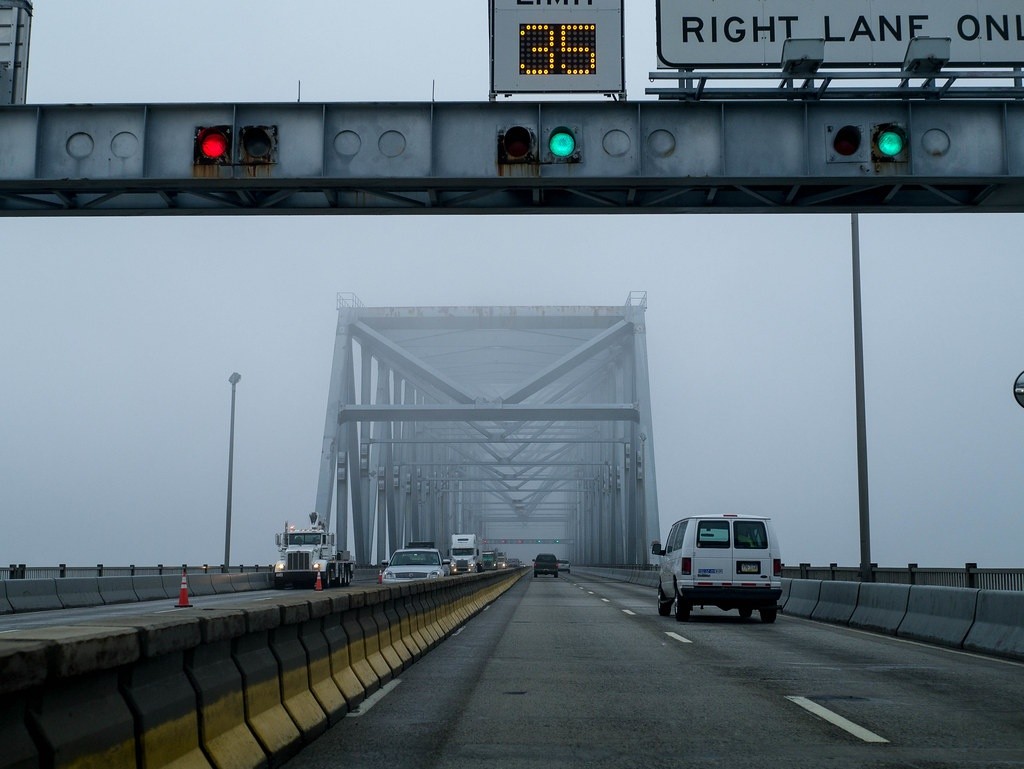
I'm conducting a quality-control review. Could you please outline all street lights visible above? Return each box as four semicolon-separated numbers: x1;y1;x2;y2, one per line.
221;371;243;573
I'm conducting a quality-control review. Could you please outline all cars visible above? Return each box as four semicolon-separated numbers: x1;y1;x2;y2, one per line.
1015;385;1024;394
557;559;572;573
380;540;451;584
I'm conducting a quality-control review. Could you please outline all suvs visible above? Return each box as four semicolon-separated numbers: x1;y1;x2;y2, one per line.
651;513;785;625
531;553;560;578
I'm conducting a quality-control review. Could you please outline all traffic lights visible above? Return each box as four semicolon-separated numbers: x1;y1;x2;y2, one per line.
193;123;280;166
824;119;910;164
497;121;584;165
488;0;626;95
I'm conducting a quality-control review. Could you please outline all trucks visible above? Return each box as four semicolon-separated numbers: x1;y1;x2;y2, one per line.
442;532;523;577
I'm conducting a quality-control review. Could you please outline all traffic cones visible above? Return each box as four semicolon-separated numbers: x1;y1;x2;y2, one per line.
377;569;383;584
173;571;194;608
313;570;323;591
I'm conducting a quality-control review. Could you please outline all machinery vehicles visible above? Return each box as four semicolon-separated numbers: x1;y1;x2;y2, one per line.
271;509;356;590
